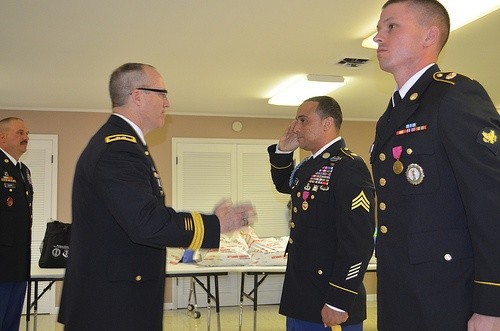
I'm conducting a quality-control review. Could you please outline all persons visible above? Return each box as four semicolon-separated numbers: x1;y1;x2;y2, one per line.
0;116;34;330
369;0;499;331
267;96;375;331
57;63;257;331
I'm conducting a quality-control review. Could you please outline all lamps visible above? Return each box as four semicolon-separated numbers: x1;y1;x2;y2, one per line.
267;75;344;106
362;0;500;53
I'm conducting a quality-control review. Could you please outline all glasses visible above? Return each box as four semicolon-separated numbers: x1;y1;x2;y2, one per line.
130;88;168;100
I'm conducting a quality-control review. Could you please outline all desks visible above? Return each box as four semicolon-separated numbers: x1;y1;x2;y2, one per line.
26;260;377;331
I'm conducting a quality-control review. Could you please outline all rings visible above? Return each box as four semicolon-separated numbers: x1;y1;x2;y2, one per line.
243;218;248;227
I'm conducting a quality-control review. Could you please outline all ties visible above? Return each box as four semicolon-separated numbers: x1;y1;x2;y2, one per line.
16;162;25;184
394;91;402;107
